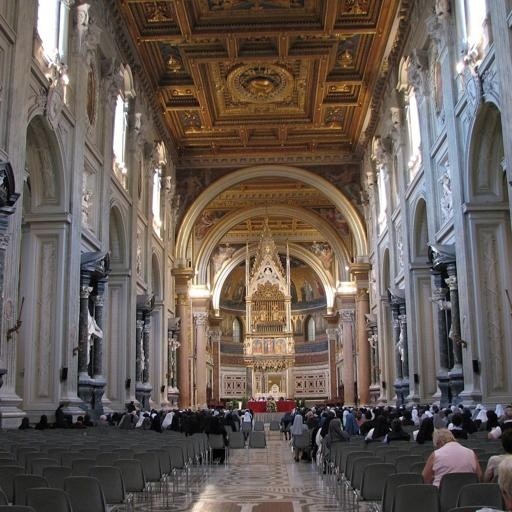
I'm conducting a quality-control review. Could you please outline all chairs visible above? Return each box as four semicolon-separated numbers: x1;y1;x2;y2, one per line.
269;403;511;511
0;404;266;512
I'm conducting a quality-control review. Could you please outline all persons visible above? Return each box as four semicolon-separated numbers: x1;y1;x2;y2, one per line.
281;402;512;512
18;404;253;465
